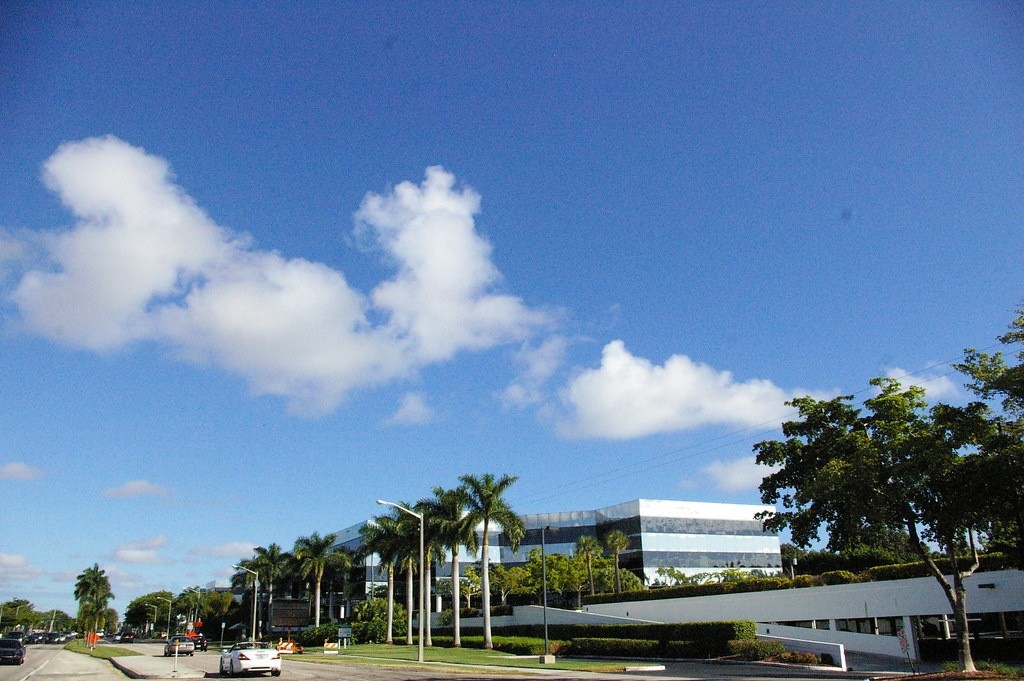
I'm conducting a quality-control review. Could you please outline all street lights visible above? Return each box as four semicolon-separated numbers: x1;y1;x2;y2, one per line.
182;587;200;631
157;597;171;641
539;525;560;663
145;604;157;623
376;500;424;663
232;565;258;641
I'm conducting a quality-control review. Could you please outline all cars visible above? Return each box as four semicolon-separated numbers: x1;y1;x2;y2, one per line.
0;639;27;665
112;634;122;641
219;641;282;677
119;633;134;643
191;636;207;652
164;636;194;657
2;631;79;645
